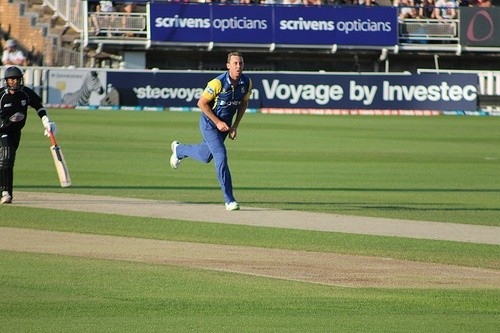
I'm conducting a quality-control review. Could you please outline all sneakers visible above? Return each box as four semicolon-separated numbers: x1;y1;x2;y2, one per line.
225;200;239;210
170;140;182;168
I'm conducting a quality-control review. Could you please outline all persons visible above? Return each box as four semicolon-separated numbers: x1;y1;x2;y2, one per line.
0;66;57;204
170;52;253;210
0;0;494;67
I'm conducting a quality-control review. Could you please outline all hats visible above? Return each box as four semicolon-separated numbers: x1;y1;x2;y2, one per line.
6;39;17;47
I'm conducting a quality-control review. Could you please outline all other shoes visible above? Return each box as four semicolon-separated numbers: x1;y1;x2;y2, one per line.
1;191;11;203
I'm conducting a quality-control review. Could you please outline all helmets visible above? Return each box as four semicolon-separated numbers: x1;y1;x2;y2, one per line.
3;67;23;79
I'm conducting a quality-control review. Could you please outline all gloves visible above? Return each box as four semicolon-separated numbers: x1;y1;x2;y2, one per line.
41;115;57;137
